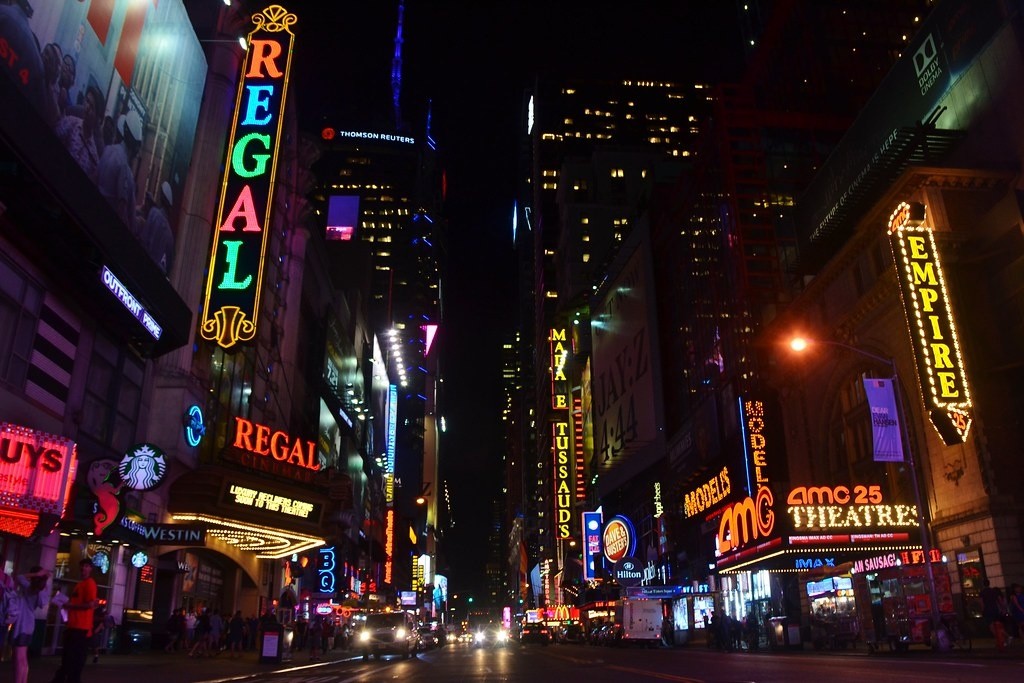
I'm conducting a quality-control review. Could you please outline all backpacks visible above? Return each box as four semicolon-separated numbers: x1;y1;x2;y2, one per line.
0;574;21;624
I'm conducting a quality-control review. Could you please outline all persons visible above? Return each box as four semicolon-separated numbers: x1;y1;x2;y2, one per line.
0;566;54;683
980;578;1024;651
59;558;115;683
710;602;773;652
164;605;352;661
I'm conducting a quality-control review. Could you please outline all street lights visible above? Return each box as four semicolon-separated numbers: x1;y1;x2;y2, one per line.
787;337;944;658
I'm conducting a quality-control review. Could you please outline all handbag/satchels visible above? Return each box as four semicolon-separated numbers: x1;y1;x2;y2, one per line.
61;628;88;644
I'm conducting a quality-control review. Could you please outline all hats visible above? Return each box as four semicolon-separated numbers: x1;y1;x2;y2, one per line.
79;558;93;567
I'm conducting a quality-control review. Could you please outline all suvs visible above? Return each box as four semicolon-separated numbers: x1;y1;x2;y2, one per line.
361;610;419;661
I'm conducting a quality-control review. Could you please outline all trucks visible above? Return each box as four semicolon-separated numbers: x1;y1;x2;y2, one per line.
616;596;664;646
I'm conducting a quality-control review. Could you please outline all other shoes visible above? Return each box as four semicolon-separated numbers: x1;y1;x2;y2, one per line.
93;655;98;664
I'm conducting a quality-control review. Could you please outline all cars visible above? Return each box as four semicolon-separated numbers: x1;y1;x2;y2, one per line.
556;626;588;644
590;622;620;645
520;623;549;647
471;623;505;648
415;619;469;653
